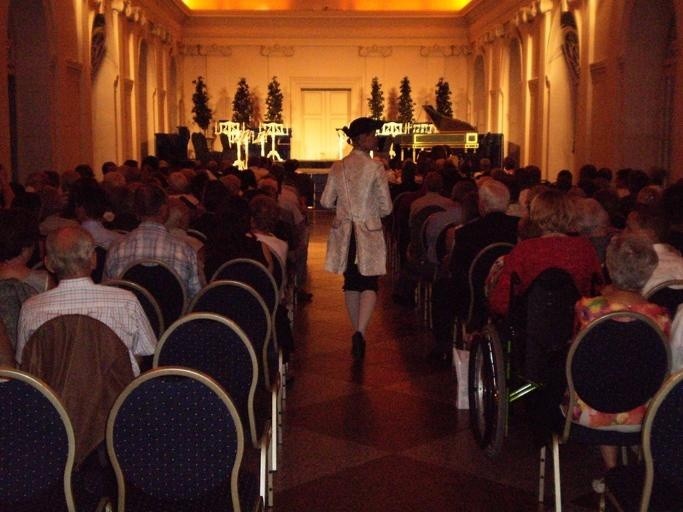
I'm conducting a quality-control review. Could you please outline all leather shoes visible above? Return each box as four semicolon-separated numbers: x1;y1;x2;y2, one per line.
352;332;366;356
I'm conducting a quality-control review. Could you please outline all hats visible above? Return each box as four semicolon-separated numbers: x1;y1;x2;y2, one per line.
350;118;383;135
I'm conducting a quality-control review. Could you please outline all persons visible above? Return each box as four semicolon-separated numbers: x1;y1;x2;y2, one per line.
447;163;680;486
3;154;313;315
370;147;496;271
319;117;393;356
16;222;158;382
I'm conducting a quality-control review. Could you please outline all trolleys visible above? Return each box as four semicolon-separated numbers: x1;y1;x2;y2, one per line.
466;267;585;455
